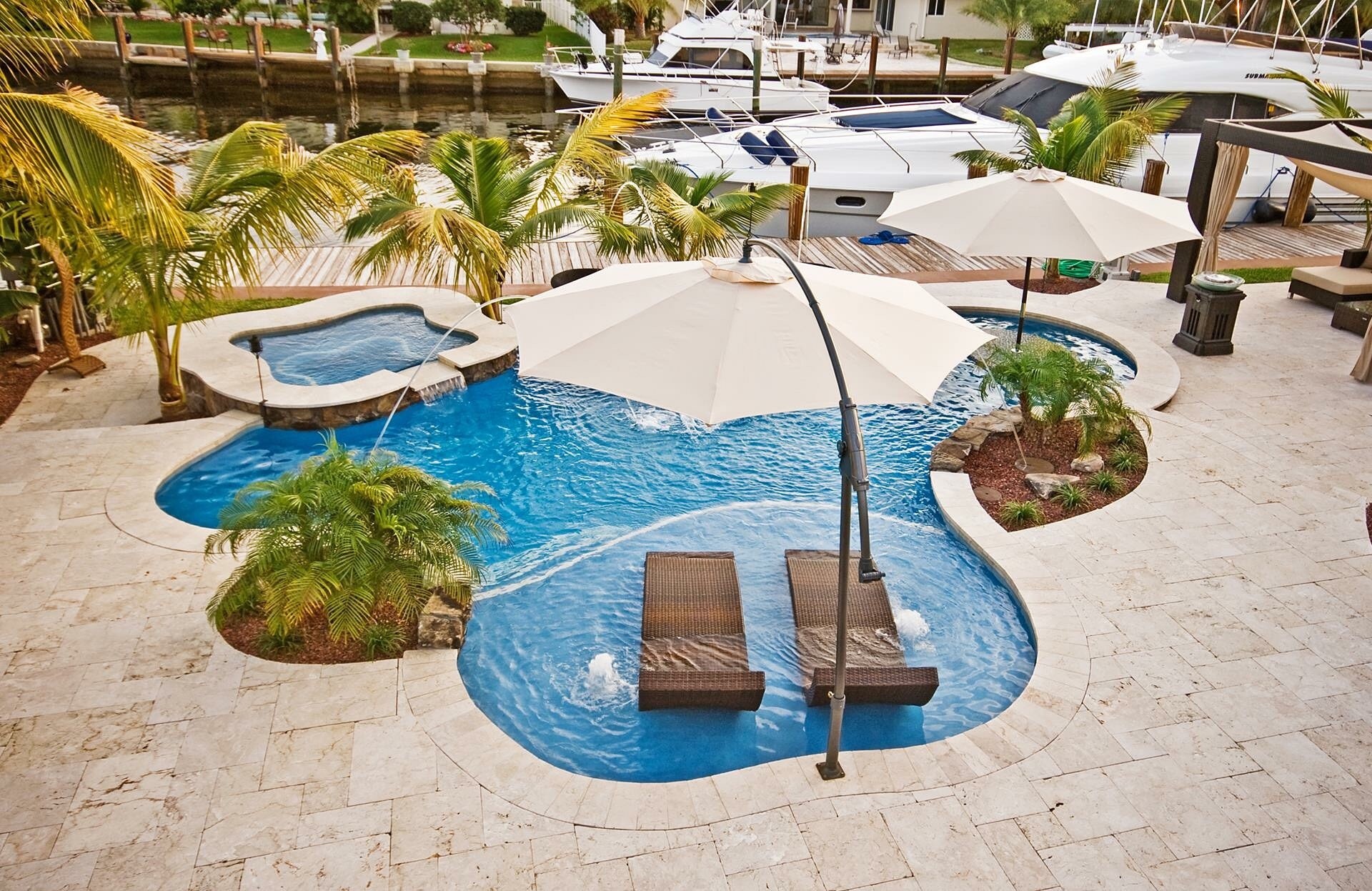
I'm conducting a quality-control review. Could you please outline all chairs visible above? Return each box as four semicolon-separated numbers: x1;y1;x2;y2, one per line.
637;551;765;710
826;43;846;64
784;10;798;29
888;35;913;59
246;31;271;55
858;21;891;43
204;28;233;52
846;39;866;64
785;548;940;708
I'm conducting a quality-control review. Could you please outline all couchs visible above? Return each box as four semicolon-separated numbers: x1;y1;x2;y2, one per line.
1289;248;1372;308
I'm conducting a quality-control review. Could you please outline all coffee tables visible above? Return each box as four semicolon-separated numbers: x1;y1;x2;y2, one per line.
1330;301;1372;335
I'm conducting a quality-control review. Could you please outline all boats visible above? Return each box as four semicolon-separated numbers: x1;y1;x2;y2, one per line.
549;18;842;116
622;21;1372;239
1042;23;1162;58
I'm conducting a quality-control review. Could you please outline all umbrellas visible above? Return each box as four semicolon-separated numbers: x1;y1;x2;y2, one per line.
877;161;1205;352
502;255;998;781
833;3;844;43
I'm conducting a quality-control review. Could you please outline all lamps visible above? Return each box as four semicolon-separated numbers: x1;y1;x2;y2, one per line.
248;335;263;355
